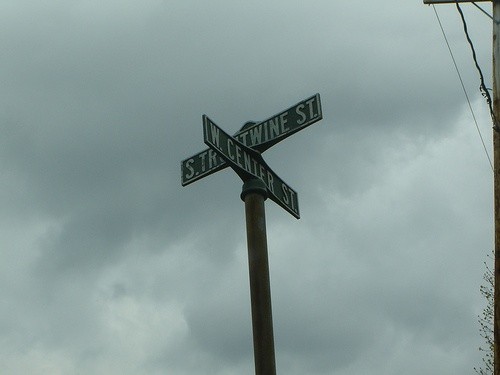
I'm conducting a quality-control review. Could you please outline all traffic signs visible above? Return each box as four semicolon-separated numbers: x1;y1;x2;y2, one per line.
202;112;300;220
179;91;323;189
